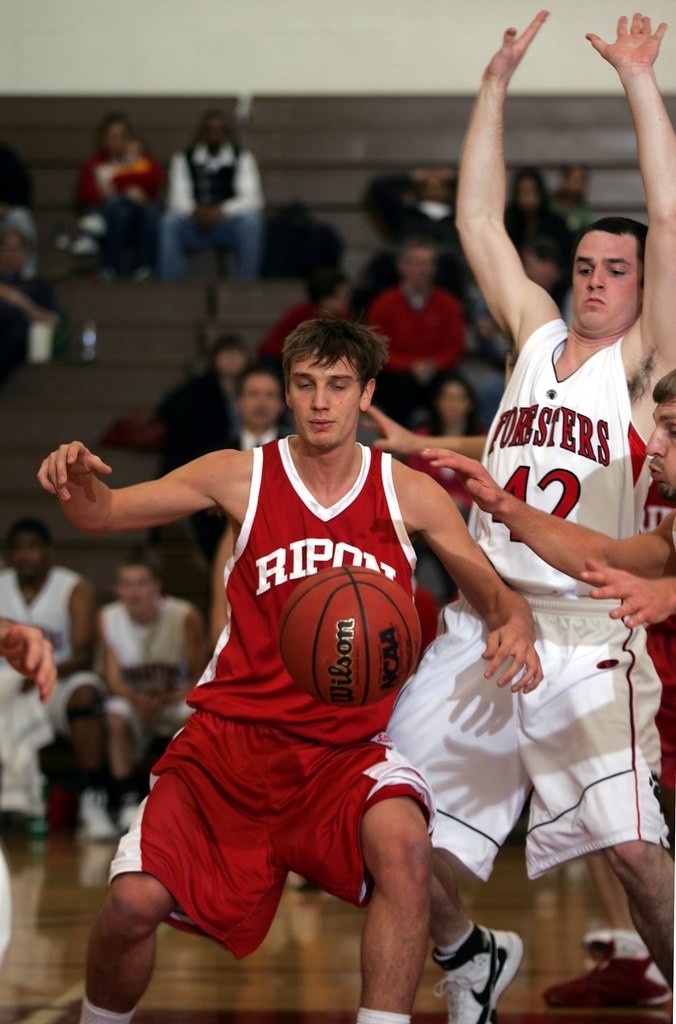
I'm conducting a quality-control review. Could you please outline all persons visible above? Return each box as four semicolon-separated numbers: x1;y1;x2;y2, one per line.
1;108;676;889
369;401;676;1009
386;10;676;1024
418;366;676;855
36;321;544;1024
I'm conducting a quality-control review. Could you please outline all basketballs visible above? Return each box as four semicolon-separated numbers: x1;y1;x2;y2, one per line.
275;565;423;710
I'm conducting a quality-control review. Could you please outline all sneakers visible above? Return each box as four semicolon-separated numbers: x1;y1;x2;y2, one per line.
431;924;524;1024
542;934;673;1006
